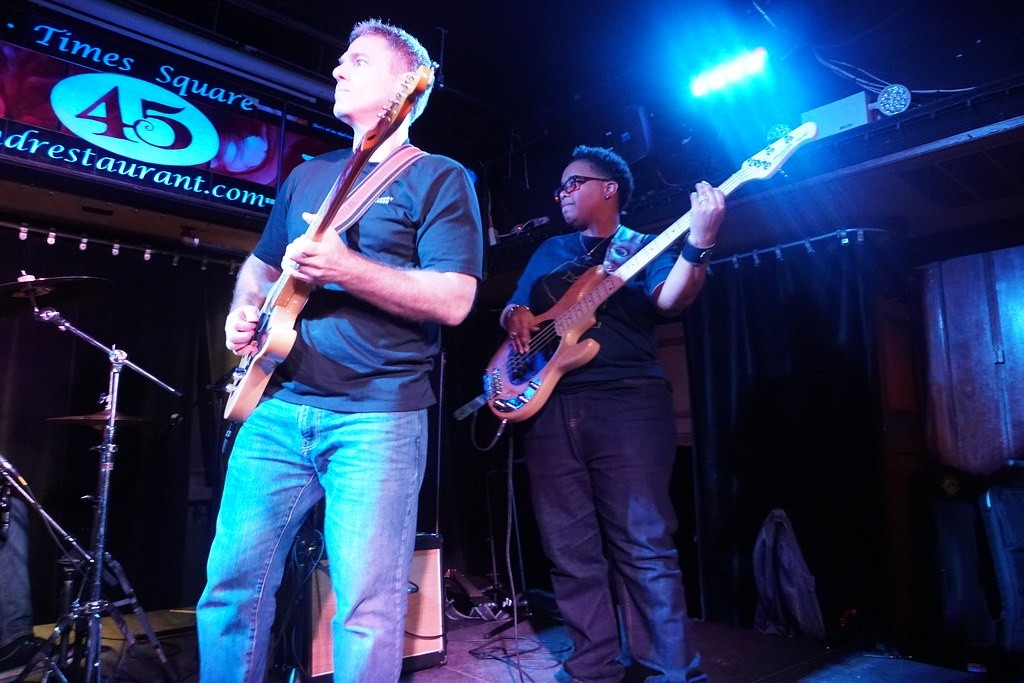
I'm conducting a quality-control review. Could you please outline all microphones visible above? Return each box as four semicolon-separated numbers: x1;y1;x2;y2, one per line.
0;455;17;476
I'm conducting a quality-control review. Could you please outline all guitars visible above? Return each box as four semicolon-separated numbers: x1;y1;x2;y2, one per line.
223;64;431;423
483;121;817;423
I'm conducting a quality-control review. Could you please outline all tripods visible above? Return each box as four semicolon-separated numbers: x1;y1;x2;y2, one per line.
482;487;560;638
11;287;181;682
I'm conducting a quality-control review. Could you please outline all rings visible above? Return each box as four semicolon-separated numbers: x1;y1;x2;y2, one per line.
509;332;517;338
294;263;301;271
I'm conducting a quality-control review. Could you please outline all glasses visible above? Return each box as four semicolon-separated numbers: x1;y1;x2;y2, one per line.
552;175;620;203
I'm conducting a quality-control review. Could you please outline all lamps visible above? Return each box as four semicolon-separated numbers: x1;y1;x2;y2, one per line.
766;83;913;146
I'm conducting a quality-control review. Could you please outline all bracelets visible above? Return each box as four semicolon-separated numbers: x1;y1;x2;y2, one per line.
507;304;529;317
682;232;717;266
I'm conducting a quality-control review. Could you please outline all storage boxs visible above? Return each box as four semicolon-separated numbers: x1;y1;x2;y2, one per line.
274;533;446;683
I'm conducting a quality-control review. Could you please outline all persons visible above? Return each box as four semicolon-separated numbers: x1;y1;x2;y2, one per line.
193;18;484;683
0;498;119;683
486;142;725;683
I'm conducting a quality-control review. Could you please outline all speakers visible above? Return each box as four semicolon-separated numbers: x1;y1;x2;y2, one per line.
274;531;449;683
1;485;28;658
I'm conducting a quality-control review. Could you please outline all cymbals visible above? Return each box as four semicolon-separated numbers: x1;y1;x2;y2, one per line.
0;276;116;299
41;408;162;433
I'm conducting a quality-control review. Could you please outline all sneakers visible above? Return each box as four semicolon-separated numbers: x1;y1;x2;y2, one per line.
0;634;75;683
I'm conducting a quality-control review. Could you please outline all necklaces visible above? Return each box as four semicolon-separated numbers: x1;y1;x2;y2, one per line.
579;224;623;260
